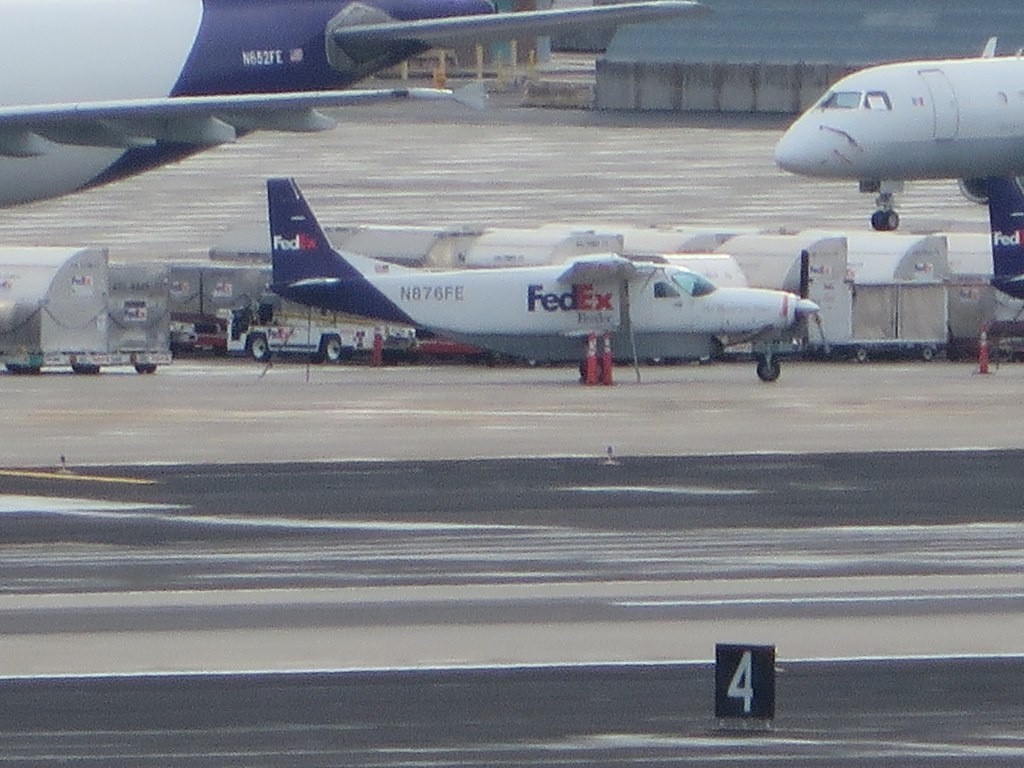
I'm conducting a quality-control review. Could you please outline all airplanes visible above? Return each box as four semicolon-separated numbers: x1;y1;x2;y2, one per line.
772;36;1024;231
264;177;820;383
0;0;712;202
